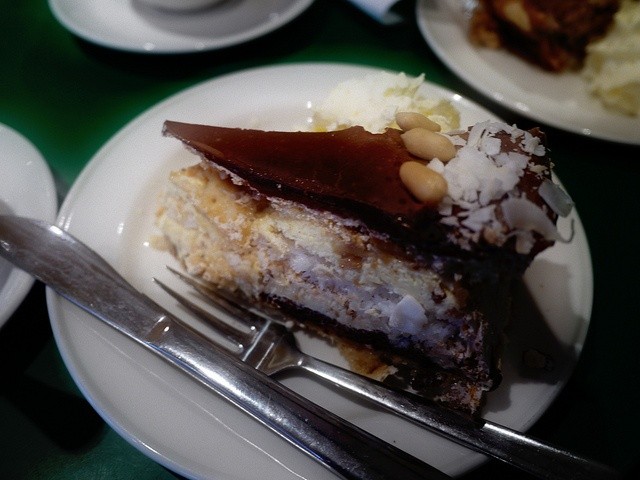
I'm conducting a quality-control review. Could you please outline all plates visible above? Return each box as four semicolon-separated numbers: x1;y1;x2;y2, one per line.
417;2;639;145
54;0;310;53
2;127;59;335
45;60;594;479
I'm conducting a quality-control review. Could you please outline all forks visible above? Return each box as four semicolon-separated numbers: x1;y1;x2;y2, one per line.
137;263;608;478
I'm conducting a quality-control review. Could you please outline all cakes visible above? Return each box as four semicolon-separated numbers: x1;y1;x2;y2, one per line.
149;71;573;422
468;0;640;117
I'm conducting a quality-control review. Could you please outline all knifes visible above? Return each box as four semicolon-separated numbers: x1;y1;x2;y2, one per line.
0;215;455;479
